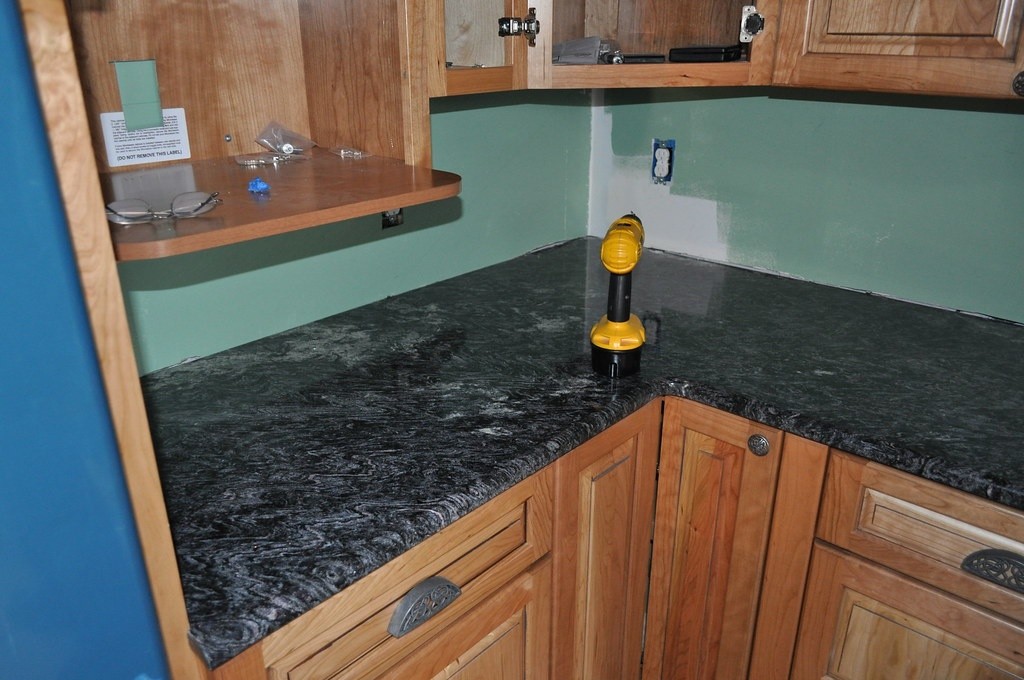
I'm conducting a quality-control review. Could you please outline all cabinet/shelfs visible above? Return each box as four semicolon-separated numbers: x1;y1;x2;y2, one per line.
63;0;1023;265
204;398;1024;679
